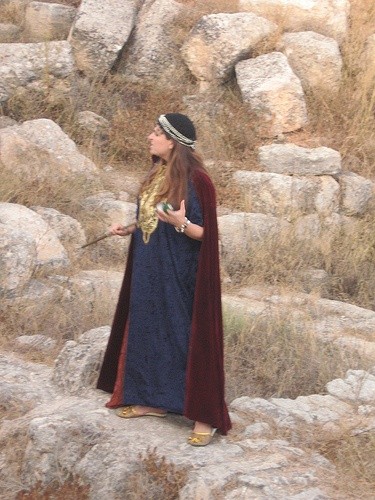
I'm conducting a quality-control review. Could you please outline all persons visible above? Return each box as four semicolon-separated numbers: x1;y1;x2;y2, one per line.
96;113;233;446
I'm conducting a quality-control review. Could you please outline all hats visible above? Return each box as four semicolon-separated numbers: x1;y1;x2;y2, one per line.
158;113;197;149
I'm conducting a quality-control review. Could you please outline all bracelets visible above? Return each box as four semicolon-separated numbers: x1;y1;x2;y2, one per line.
174;217;190;233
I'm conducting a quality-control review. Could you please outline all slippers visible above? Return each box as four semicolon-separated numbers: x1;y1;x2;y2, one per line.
116;405;168;418
187;428;218;446
